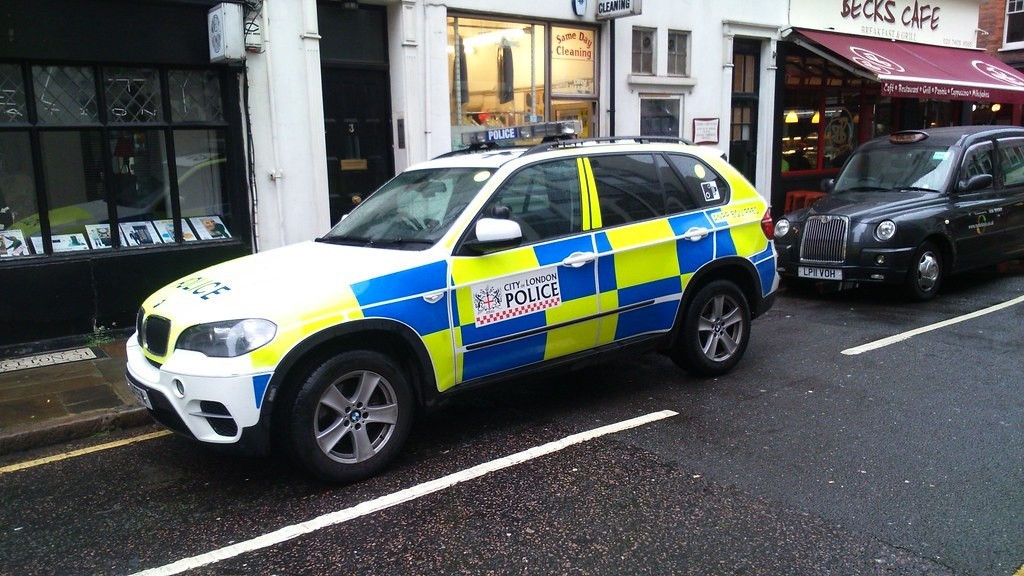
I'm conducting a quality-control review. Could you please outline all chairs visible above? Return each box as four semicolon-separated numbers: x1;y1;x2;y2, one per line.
544;165;685;236
968;161;987;178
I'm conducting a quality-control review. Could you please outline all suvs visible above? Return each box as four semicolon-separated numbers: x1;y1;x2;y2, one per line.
6;139;391;256
772;123;1023;301
122;119;780;483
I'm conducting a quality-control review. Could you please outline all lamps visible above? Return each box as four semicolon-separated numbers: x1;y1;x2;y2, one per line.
785;110;798;123
811;111;819;123
